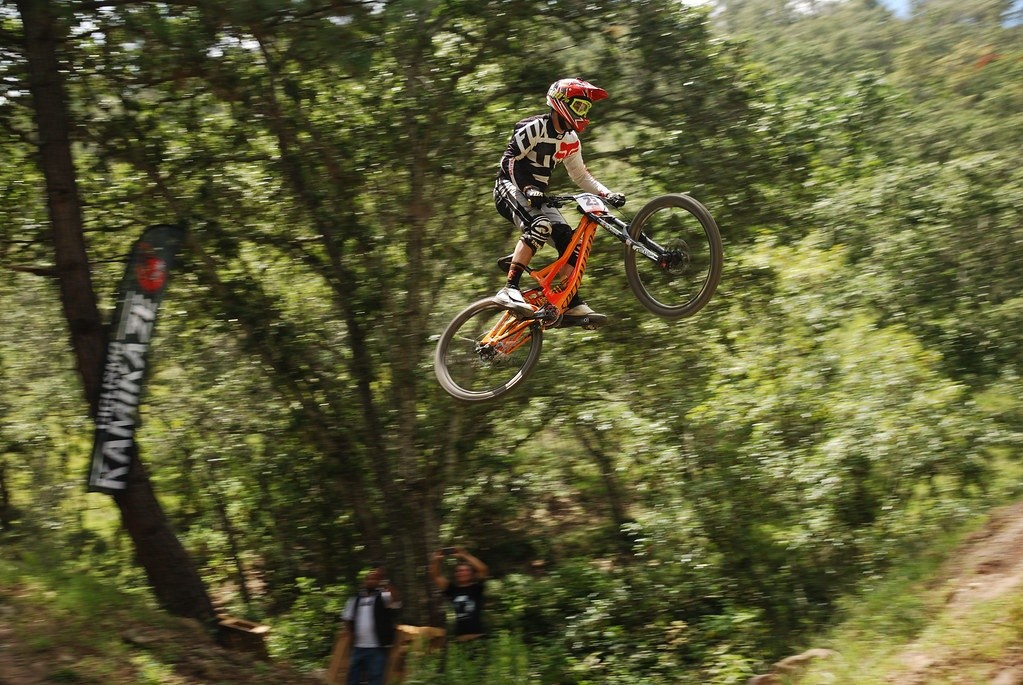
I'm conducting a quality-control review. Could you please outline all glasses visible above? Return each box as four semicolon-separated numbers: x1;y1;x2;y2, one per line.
568;98;592;118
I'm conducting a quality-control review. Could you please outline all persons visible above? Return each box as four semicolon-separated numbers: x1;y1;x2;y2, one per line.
340;566;402;685
430;545;488;661
492;77;626;328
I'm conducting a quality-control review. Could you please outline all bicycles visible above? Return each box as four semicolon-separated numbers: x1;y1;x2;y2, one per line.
433;188;722;405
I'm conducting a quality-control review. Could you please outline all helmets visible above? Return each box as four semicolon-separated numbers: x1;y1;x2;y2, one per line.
546;78;608;133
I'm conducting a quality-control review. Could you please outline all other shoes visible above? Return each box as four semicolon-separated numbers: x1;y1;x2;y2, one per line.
563;303;608;325
493;284;534;318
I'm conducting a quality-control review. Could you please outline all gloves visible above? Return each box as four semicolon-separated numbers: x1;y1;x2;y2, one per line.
524;187;545;209
606;192;626;207
